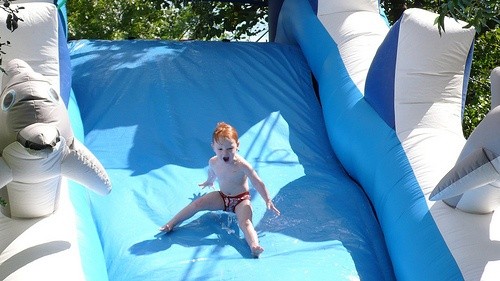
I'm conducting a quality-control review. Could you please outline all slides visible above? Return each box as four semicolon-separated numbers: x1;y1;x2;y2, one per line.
68;40;394;280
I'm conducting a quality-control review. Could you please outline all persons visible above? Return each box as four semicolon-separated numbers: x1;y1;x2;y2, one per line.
159;122;280;258
